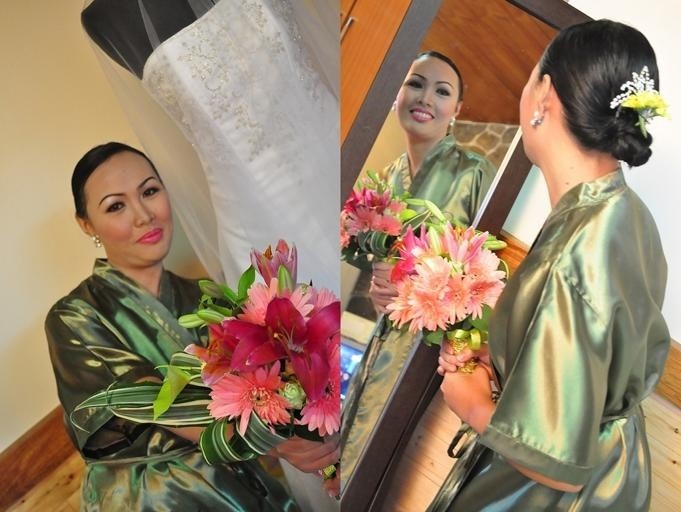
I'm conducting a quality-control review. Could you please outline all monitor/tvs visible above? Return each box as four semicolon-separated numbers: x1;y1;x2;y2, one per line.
340;335;368;401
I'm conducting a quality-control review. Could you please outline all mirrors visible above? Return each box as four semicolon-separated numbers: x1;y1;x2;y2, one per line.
339;1;561;507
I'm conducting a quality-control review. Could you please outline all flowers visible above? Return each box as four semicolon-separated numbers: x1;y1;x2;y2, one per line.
69;238;340;499
384;199;509;375
339;167;425;265
620;89;673;139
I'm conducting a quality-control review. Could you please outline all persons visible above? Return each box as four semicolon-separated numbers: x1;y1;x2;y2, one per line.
424;19;671;512
340;50;497;503
44;141;340;512
80;0;340;301
